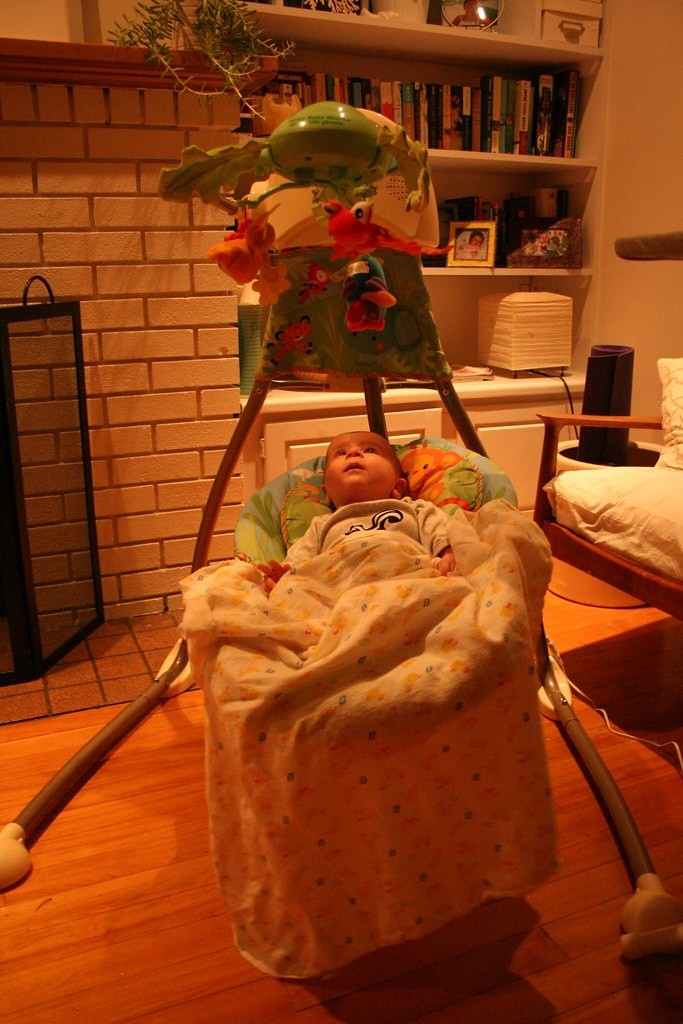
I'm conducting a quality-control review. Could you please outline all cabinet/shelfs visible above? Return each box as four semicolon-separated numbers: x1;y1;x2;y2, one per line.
253;391;584;521
216;0;605;414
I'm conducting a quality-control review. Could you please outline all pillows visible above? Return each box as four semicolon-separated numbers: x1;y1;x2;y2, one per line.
654;358;683;472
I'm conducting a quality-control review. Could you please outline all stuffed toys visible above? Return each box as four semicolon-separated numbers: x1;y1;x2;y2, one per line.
207;202;290;305
376;122;430;213
320;197;395;333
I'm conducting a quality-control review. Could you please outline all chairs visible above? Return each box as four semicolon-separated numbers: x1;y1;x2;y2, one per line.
532;413;683;622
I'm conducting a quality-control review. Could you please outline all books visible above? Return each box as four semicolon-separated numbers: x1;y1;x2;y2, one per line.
424;186;568;265
238;63;582;157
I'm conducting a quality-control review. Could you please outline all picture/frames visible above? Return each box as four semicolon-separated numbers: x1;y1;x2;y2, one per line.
446;220;498;268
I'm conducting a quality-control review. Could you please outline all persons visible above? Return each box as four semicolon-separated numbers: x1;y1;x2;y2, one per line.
257;430;456;594
451;0;492;26
459;231;486;259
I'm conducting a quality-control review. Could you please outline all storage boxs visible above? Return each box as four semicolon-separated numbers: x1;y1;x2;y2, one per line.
542;0;604;48
